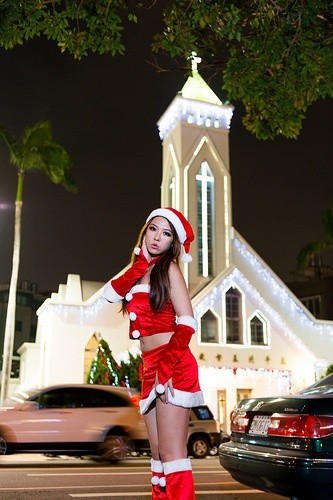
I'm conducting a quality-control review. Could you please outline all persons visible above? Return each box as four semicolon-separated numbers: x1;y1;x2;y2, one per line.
104;208;204;500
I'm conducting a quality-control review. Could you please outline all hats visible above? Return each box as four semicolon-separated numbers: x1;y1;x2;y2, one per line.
146;207;194;263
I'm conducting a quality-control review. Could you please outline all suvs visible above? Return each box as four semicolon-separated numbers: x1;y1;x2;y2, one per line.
0;383;141;463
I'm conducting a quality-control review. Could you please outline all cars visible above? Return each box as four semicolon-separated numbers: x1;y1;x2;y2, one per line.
218;373;333;500
132;405;219;459
